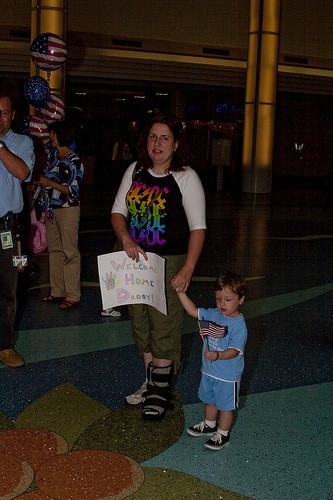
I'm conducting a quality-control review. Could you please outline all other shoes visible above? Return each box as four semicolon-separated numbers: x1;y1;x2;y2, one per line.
0;349;25;367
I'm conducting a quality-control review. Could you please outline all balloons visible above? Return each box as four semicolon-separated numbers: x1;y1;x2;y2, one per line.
30;33;68;71
19;76;65;146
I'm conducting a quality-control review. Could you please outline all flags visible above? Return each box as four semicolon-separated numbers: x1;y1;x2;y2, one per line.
199;321;225;338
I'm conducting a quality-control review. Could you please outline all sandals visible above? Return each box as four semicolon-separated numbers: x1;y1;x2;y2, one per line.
58;299;79;310
101;308;121;316
42;296;65;302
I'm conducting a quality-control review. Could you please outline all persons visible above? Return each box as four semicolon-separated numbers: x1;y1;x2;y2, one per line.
111;116;207;419
36;121;85;310
0;93;36;368
100;239;123;317
171;272;248;449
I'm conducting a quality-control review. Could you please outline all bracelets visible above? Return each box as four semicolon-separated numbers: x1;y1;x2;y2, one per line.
214;351;219;361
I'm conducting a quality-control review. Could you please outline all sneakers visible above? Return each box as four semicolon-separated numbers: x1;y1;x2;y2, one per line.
204;431;230;450
187;422;218;436
124;382;149;404
